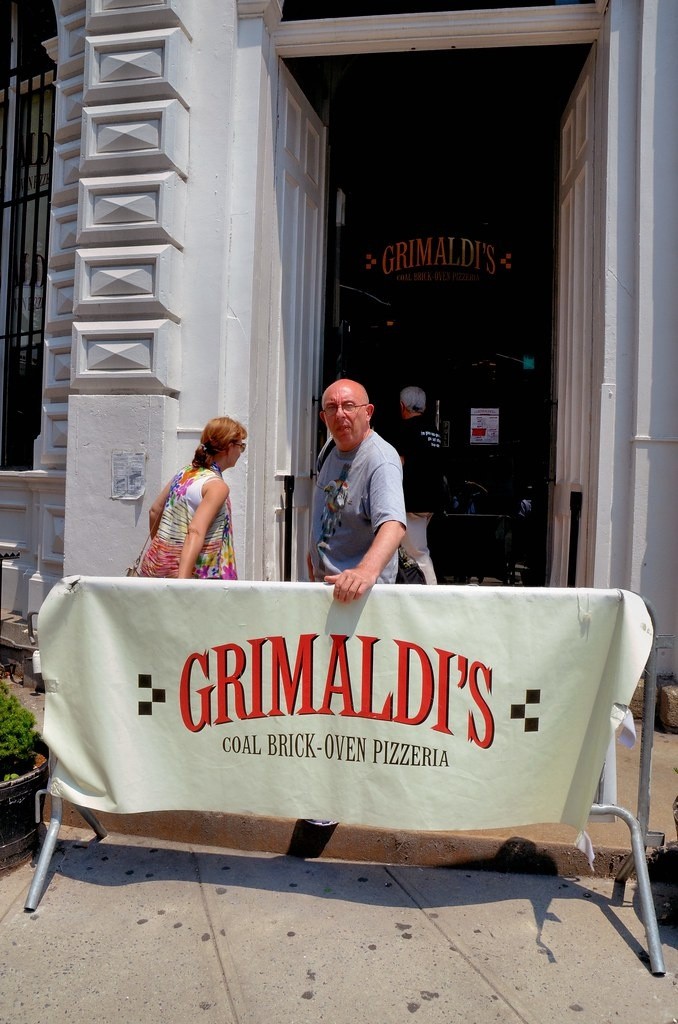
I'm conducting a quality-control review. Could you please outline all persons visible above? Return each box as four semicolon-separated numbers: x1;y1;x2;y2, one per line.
303;379;407;826
397;386;442;586
140;418;247;579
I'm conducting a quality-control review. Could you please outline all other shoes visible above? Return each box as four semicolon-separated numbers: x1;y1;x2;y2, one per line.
305;819;337;827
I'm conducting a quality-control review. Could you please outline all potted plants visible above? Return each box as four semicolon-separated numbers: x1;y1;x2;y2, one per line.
0;681;49;869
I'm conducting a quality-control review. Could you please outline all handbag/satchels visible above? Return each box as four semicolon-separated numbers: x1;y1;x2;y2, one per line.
393;554;427;585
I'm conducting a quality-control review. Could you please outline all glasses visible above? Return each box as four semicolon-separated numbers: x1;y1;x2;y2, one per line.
323;402;369;416
231;441;247;452
